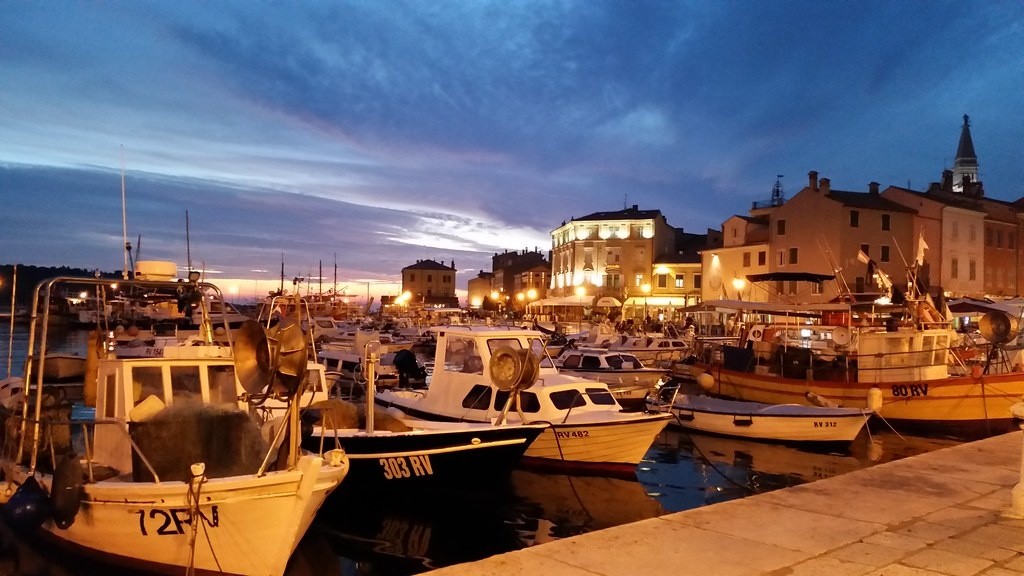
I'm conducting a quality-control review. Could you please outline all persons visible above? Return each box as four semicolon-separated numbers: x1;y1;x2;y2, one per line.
618;311;699;335
393;349;417;388
884;312;898;332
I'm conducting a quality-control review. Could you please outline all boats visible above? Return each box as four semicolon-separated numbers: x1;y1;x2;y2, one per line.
127;210;249;328
567;333;690;361
638;384;882;448
679;294;1024;433
371;325;681;480
274;346;554;556
254;253;363;326
0;169;349;576
551;347;674;403
309;315;419;366
